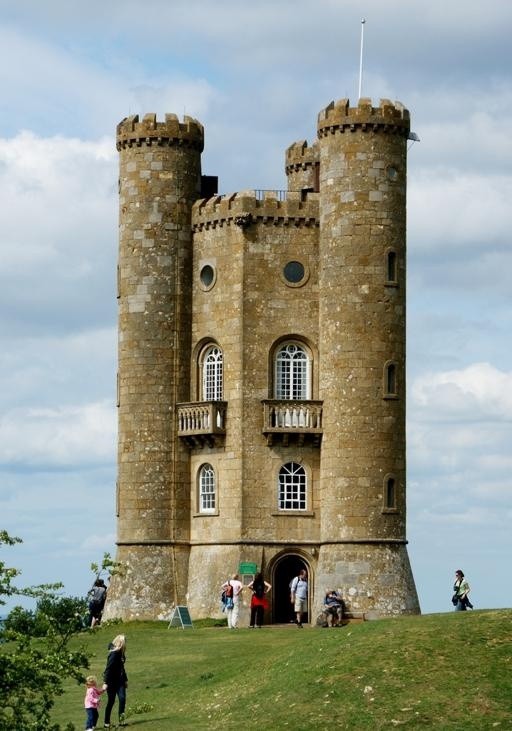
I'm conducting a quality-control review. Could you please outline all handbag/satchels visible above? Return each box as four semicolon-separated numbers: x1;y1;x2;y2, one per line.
223;579;234;598
452;595;458;606
288;576;299;593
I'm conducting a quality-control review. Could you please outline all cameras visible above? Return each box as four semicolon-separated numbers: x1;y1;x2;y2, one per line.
453;585;459;591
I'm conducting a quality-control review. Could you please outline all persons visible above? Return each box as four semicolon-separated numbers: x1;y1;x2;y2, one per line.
289;570;308;629
83;676;106;731
102;634;129;730
222;574;244;630
453;570;470;612
85;581;107;627
89;580;106;631
324;590;345;628
246;572;272;628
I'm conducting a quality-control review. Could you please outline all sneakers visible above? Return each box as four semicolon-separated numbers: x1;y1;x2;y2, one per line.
225;620;345;630
